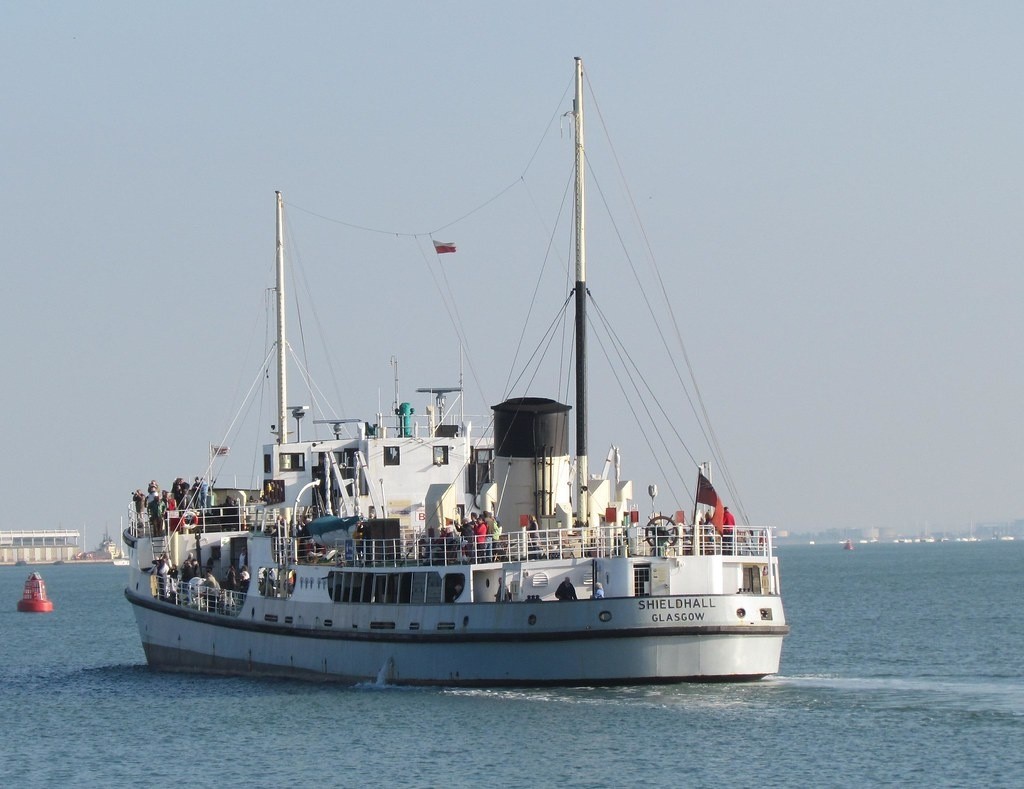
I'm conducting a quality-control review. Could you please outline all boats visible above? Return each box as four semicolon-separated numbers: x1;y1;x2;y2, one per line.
123;53;790;685
306;515;359;546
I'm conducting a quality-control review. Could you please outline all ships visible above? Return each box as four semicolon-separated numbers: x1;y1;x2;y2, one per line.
1;515;130;567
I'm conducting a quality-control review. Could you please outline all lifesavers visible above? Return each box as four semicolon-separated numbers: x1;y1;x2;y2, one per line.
307;538;327;557
462;540;472;562
181;511;198;529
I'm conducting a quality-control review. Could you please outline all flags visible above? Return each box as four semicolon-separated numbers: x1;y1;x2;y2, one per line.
697;473;723;537
432;240;456;254
211;445;230;456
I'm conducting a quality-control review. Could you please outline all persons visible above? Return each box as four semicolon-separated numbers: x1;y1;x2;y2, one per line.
238;547;248;576
290;513;375;563
200;477;208;513
226;566;236;606
445;526;453;564
144;475;191;537
152;551;200;606
192;476;201;511
238;565;250;592
274;514;286;556
722;507;734;555
528;513;540;561
199;569;220;612
455;518;473;564
468;511;499;563
594;582;604;599
259;568;276;597
133;488;147;528
555;577;577;600
